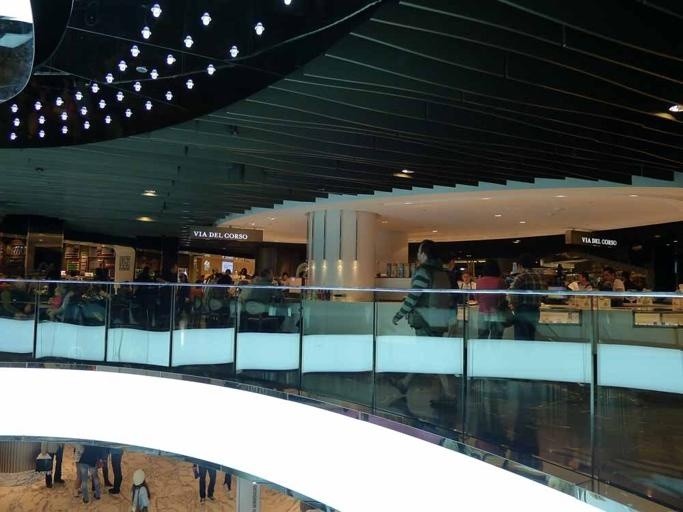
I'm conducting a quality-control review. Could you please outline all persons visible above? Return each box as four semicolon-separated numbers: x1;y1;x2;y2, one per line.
222;472;234;500
130;469;150;512
0;267;291;330
34;440;124;504
192;463;216;505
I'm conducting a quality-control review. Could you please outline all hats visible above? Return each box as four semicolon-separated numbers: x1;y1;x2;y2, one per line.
133;469;145;486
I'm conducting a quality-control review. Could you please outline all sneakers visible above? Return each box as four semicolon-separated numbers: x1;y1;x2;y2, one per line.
200;496;215;504
430;396;457;406
389;376;408;394
74;480;120;503
46;479;65;488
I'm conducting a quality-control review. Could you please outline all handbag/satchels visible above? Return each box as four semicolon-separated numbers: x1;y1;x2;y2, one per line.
497;302;514;327
35;453;52;473
